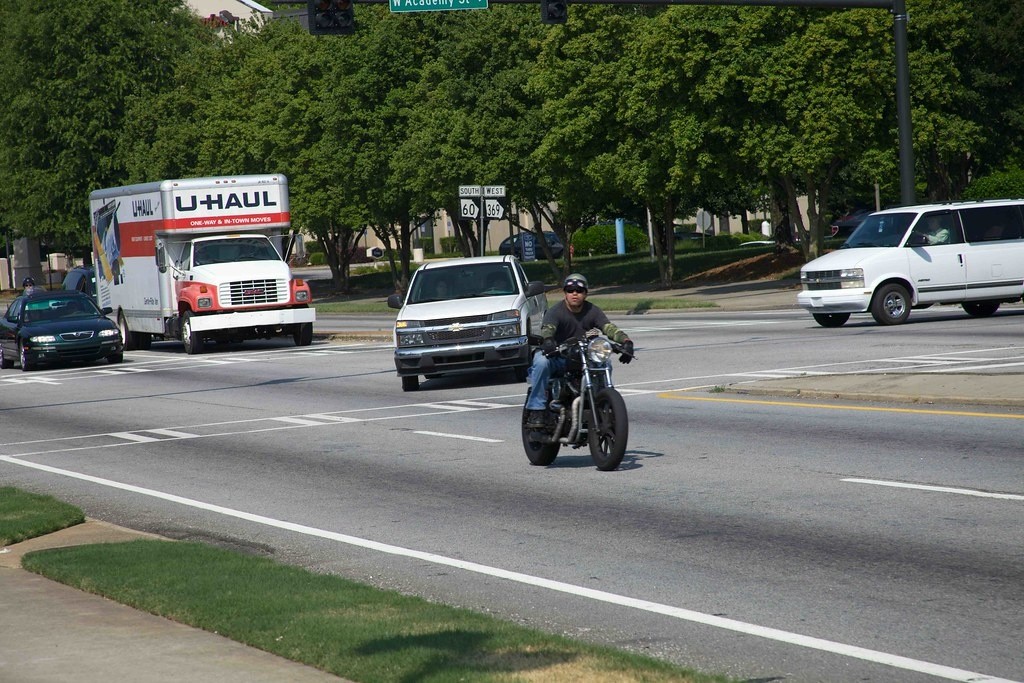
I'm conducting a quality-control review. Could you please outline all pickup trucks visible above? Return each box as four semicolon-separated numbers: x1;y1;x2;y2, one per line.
386;253;550;392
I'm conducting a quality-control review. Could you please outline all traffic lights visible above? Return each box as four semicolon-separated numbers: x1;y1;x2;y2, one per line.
307;0;354;36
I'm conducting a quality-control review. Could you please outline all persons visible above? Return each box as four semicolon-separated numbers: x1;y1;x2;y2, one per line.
916;217;950;245
526;272;634;427
17;277;37;297
435;279;448;297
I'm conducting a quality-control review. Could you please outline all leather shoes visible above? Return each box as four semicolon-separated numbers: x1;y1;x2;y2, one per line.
523;411;545;429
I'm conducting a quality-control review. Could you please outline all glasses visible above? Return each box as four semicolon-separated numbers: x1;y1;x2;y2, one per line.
25;285;32;287
564;288;585;294
565;279;586;287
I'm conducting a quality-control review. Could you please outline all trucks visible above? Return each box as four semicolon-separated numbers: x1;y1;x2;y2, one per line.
88;171;316;355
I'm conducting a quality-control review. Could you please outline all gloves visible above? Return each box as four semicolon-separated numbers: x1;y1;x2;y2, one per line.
540;338;557;352
619;341;634;364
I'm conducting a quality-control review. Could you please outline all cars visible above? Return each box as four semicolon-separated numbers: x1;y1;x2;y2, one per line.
58;266;96;304
0;287;124;371
499;230;565;260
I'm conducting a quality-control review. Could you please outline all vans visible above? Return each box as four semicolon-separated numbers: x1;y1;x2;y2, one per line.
796;197;1024;326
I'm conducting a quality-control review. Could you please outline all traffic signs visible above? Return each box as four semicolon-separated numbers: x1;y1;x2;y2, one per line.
389;0;488;13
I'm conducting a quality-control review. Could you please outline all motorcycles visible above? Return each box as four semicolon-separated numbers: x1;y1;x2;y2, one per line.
520;335;639;471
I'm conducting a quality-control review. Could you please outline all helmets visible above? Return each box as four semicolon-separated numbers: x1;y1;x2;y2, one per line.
563;274;588;293
22;277;35;287
